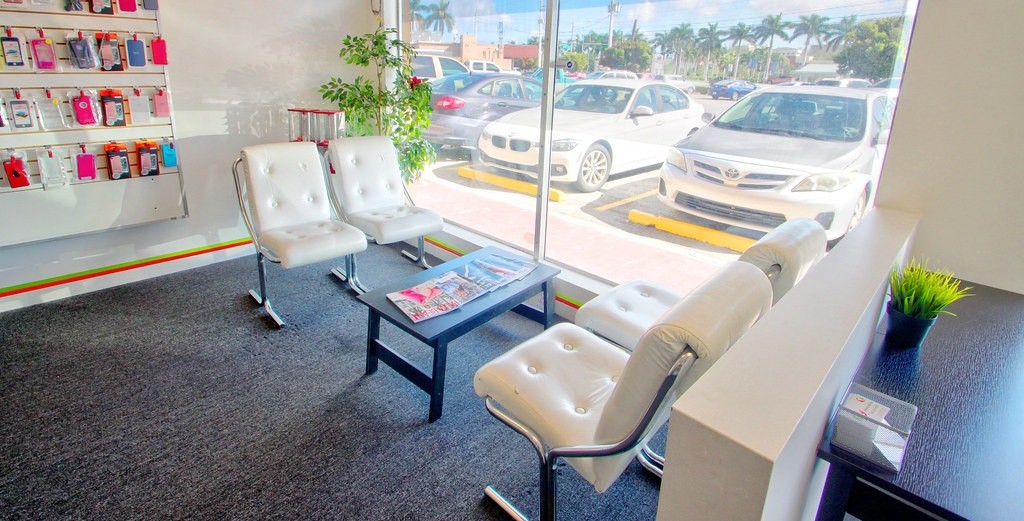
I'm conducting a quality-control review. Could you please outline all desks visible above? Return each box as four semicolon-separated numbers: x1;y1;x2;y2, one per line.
356;246;563;422
815;270;1024;521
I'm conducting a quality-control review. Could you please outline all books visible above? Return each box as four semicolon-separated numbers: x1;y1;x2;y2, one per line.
385;253;539;324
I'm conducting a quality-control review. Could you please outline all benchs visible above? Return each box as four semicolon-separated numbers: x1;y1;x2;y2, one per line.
234;135;445;329
474;214;829;521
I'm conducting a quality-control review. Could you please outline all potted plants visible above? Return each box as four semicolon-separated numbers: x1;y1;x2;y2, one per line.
885;257;974;348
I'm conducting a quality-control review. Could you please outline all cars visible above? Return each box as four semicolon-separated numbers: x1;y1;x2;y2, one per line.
409;55;705;193
657;75;902;247
